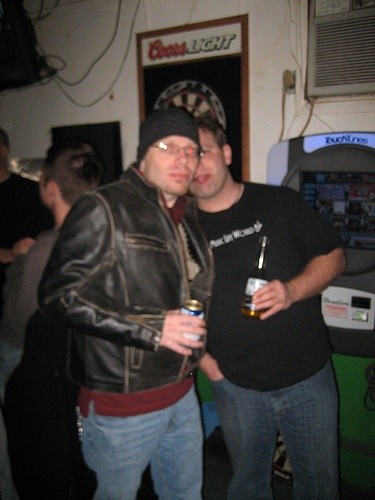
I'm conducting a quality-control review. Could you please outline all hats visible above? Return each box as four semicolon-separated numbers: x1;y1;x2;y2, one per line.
139;108;200;148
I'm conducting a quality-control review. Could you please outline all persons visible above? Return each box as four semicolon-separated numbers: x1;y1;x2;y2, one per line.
0;127;56;293
184;114;346;500
0;139;103;500
0;403;20;500
35;105;213;500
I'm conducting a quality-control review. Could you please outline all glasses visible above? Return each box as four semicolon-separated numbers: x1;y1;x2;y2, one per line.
148;141;200;158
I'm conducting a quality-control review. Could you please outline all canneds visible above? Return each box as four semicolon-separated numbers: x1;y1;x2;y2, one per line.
180;299;205;341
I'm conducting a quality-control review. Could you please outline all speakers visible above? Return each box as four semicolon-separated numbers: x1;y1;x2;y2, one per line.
0;0;40;92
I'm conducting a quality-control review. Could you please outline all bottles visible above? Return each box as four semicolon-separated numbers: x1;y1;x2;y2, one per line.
242;234;276;319
